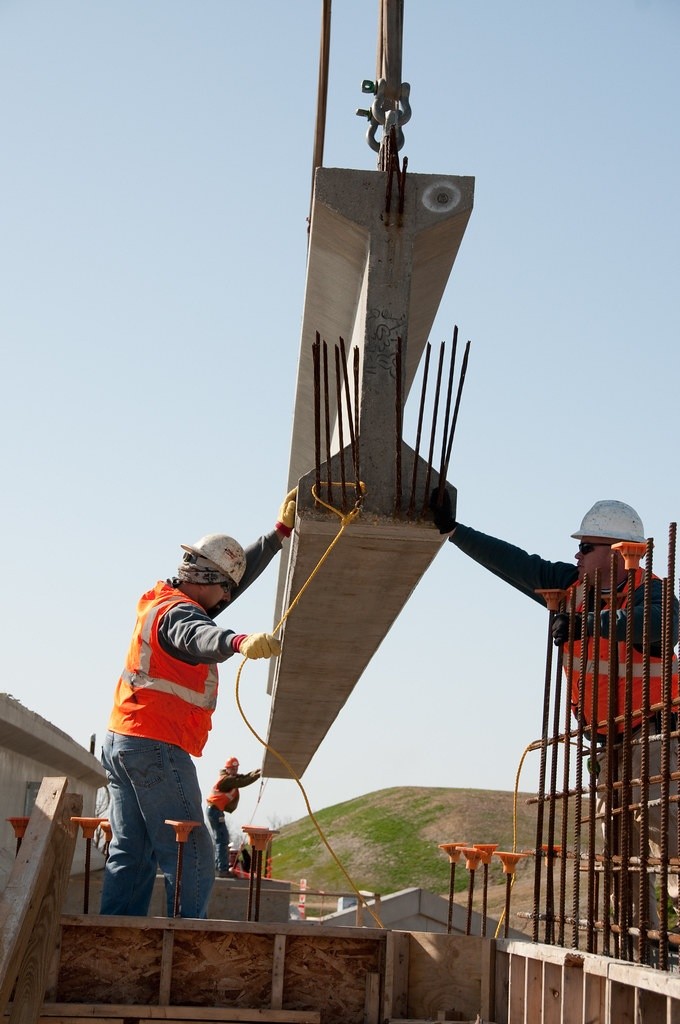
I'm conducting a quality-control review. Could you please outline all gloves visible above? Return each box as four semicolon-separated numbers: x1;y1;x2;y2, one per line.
274;485;298;538
234;633;282;659
550;613;590;646
431;487;457;534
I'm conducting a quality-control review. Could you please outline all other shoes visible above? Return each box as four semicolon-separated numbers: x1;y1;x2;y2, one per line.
215;869;234;877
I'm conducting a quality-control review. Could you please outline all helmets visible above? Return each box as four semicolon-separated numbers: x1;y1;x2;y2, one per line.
225;757;238;768
571;501;647;542
181;533;247;585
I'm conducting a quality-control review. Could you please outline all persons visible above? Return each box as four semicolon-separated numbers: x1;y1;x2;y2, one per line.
448;500;680;965
228;842;251;880
98;485;298;918
207;757;261;878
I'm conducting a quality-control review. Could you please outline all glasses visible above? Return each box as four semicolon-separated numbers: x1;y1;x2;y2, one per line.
578;542;613;555
